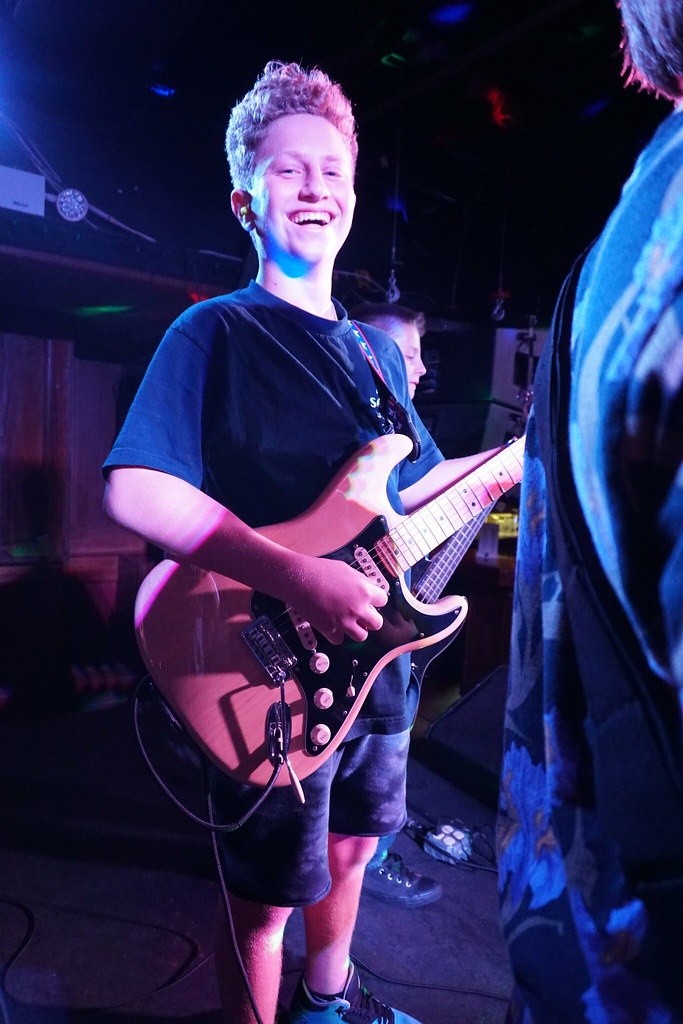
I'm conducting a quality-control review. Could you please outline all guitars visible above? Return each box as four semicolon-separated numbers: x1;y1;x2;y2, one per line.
126;428;526;810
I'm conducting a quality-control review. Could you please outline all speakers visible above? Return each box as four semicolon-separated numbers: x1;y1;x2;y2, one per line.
412;329;550;505
422;663;508;812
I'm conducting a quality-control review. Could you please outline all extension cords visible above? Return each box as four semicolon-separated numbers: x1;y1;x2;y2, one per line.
425;824;473;865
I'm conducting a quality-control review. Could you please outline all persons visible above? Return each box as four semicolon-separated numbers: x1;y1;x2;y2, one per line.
98;53;461;1024
342;300;485;911
490;2;683;1022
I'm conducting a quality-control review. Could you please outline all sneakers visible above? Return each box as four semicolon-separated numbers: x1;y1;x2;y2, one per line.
362;851;442;907
289;960;422;1024
